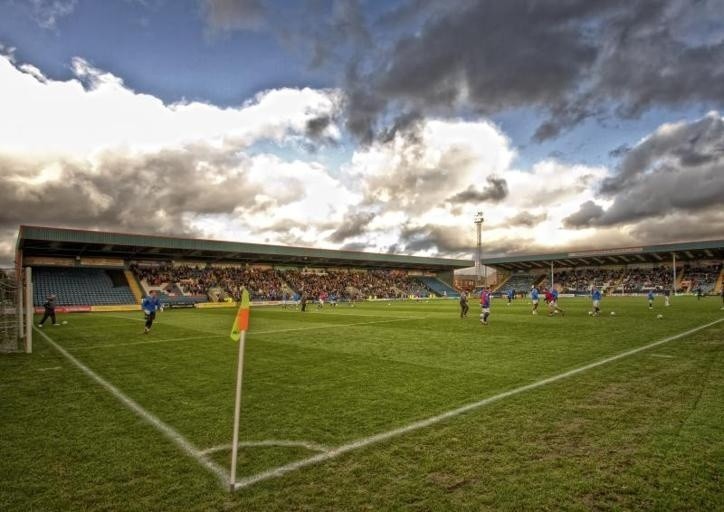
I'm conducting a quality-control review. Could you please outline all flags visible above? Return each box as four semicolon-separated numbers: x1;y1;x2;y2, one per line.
230;287;250;342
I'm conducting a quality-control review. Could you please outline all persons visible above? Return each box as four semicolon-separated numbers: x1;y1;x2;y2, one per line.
143;290;164;333
136;265;723;326
38;294;61;330
